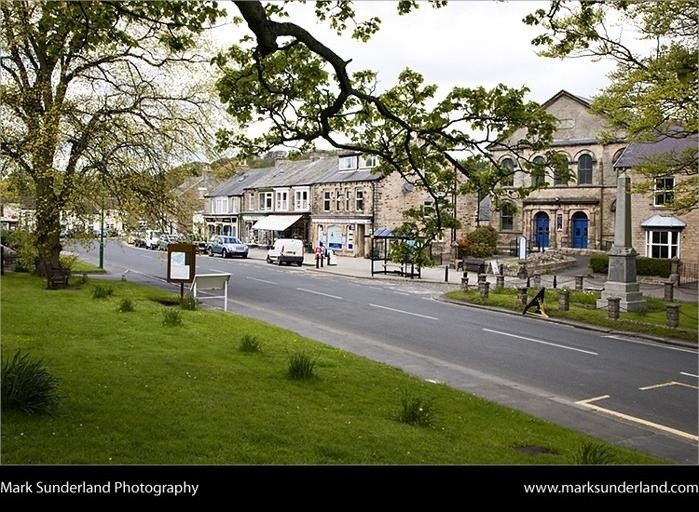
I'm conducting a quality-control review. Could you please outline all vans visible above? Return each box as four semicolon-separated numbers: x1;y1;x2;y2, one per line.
265;239;305;266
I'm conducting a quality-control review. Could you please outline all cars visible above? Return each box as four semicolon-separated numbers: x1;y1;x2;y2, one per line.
60;228;119;238
128;225;205;253
206;234;249;260
